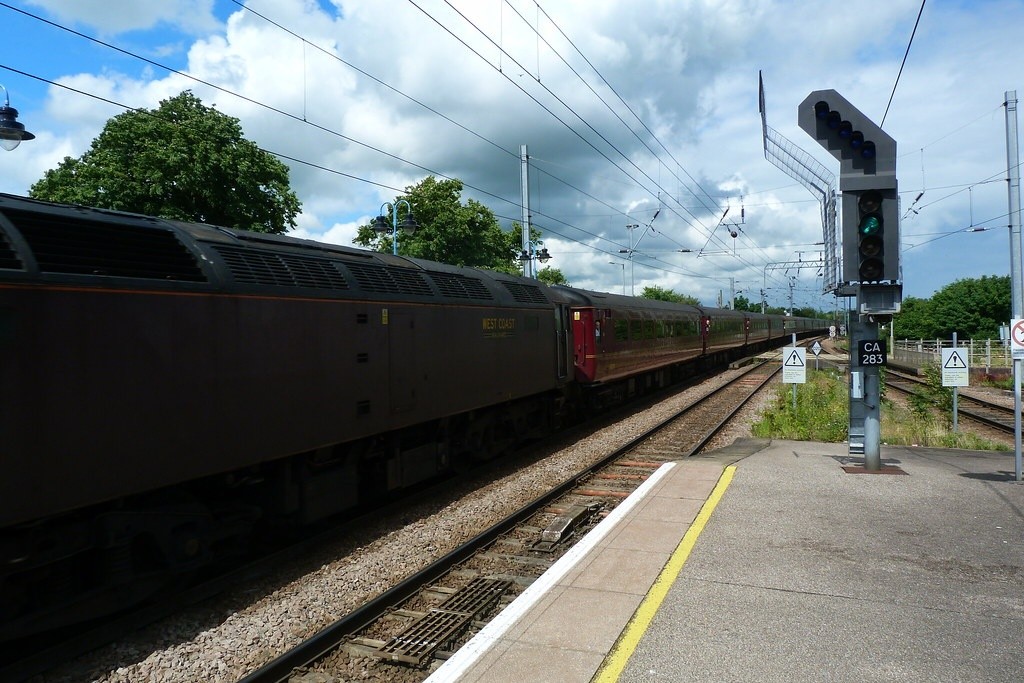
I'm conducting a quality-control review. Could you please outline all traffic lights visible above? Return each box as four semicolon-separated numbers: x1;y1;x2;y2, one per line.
858;191;884;280
814;100;875;157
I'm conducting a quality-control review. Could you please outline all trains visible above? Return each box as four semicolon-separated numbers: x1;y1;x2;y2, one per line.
1;190;844;643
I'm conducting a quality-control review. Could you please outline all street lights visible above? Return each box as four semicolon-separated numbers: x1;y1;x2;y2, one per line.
0;82;35;151
609;261;625;296
370;199;421;255
517;239;552;280
